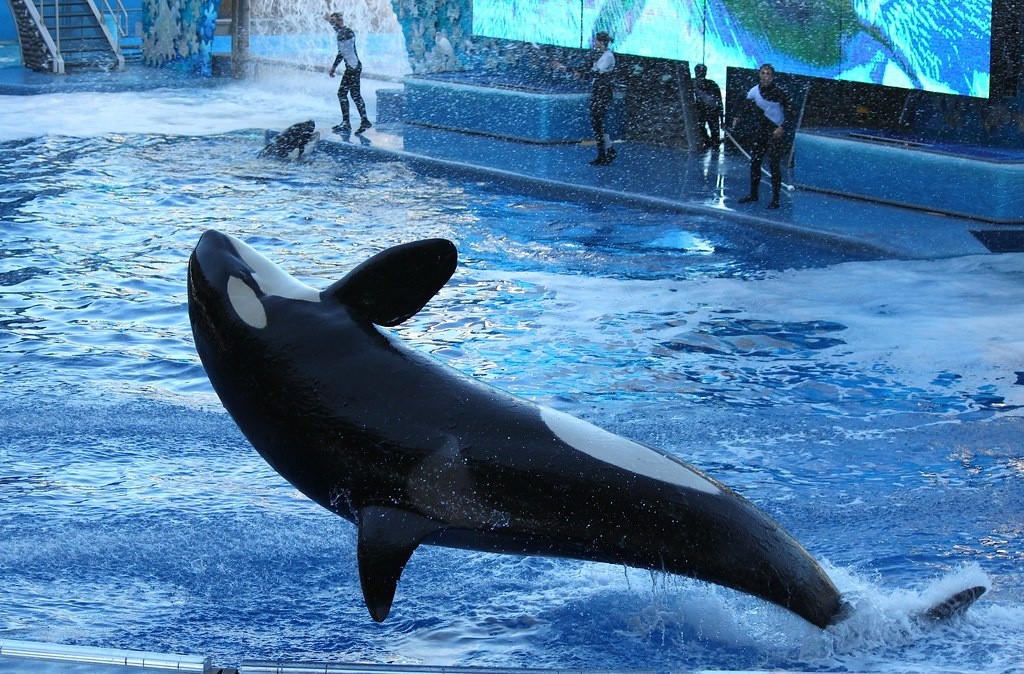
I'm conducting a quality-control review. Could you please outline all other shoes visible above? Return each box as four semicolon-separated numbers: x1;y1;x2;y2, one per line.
355;121;372;135
333;122;352;133
590;151;617;166
766;201;780;209
711;148;720;157
738;194;759;203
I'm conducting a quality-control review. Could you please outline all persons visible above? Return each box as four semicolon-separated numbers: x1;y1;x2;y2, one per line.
689;64;723;154
732;64;787;208
325;11;372;134
555;31;617;166
